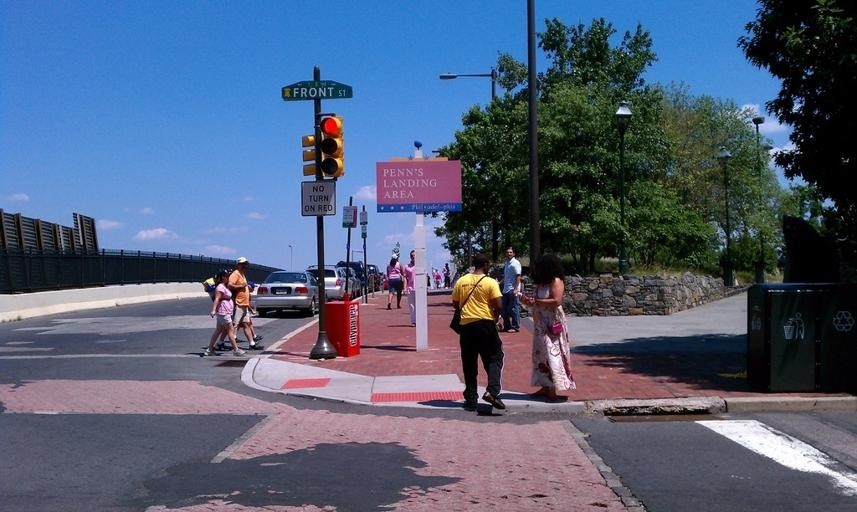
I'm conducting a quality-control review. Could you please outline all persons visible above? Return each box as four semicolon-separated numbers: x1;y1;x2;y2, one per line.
519;255;577;403
214;257;264;351
230;280;263;343
204;269;245;356
382;249;452;327
452;254;505;411
497;245;522;333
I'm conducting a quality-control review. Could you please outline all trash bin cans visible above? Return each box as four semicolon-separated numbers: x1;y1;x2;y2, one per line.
746;282;857;394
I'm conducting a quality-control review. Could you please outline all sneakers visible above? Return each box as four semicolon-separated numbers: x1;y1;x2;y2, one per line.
507;328;520;332
204;335;265;356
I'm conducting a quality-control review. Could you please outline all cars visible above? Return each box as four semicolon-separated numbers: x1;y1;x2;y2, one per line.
254;271;327;316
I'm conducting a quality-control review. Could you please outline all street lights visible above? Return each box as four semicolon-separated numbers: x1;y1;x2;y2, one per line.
610;99;633;276
714;146;734;290
751;117;766;285
438;68;501;264
288;244;293;272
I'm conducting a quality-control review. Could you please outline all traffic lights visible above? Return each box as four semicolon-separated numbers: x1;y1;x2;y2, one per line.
302;134;316;177
321;114;344;180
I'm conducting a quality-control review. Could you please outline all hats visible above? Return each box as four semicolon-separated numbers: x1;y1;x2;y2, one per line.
391;253;397;259
236;257;248;265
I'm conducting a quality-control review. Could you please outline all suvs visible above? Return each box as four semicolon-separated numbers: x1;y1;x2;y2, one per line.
336;260;385;299
304;265;352;301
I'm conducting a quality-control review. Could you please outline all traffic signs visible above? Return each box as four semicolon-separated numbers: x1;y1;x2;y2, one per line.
377;160;462;211
280;80;354;101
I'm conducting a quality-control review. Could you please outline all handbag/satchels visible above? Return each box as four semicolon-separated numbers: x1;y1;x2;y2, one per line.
450;307;461;334
550;322;562;335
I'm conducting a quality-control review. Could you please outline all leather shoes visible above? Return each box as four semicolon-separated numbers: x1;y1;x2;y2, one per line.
483;392;506;409
